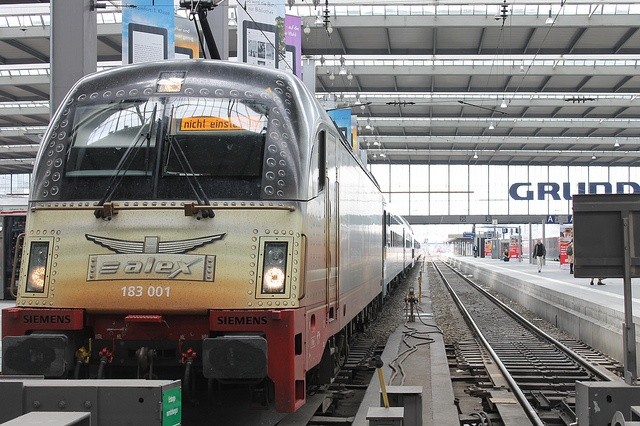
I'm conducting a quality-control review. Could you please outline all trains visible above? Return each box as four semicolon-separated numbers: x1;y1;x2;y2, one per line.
2;0;421;413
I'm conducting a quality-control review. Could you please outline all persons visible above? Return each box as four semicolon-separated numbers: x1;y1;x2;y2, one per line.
590;277;606;286
472;244;479;258
503;248;510;262
532;238;547;272
566;239;574;274
